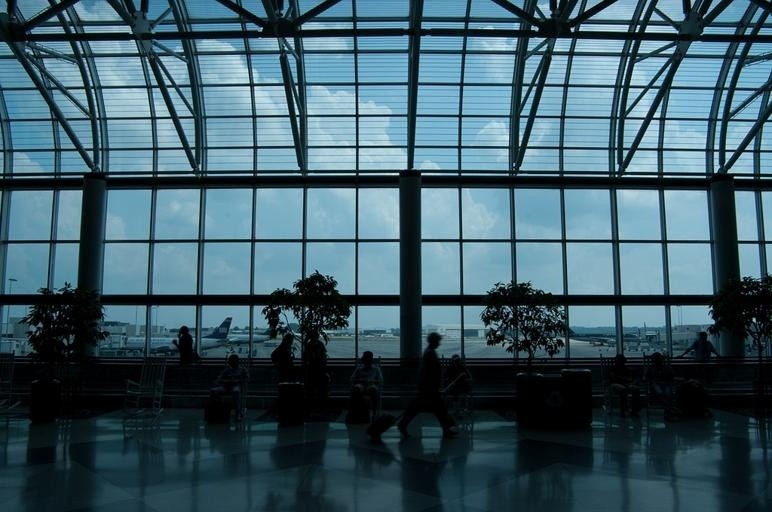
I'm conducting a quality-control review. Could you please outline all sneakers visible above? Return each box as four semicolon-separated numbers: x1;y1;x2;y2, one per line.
344;415;357;424
443;429;458;437
397;424;411;437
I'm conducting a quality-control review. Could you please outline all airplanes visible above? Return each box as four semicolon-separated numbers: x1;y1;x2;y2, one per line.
63;317;276;357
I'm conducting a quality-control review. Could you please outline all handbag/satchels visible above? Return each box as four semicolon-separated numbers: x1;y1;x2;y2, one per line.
664;407;679;422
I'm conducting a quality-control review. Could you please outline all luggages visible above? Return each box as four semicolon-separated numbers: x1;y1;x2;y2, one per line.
277;381;306;428
679;379;712;417
366;407;407;437
204;397;230;424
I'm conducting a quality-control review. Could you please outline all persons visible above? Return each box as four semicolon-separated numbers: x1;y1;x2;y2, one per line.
207;353;250;423
676;331;721;361
171;325;194;363
270;333;298;381
348;350;382;422
283;327;329;389
643;352;676;416
396;331;460;439
432;353;474;402
121;332;129;347
605;353;642;418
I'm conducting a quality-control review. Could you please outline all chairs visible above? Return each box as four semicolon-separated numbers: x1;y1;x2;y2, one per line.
599;351;673;420
215;352;251;418
0;350;16;409
441;354;473;430
353;355;382;421
122;351;167;418
